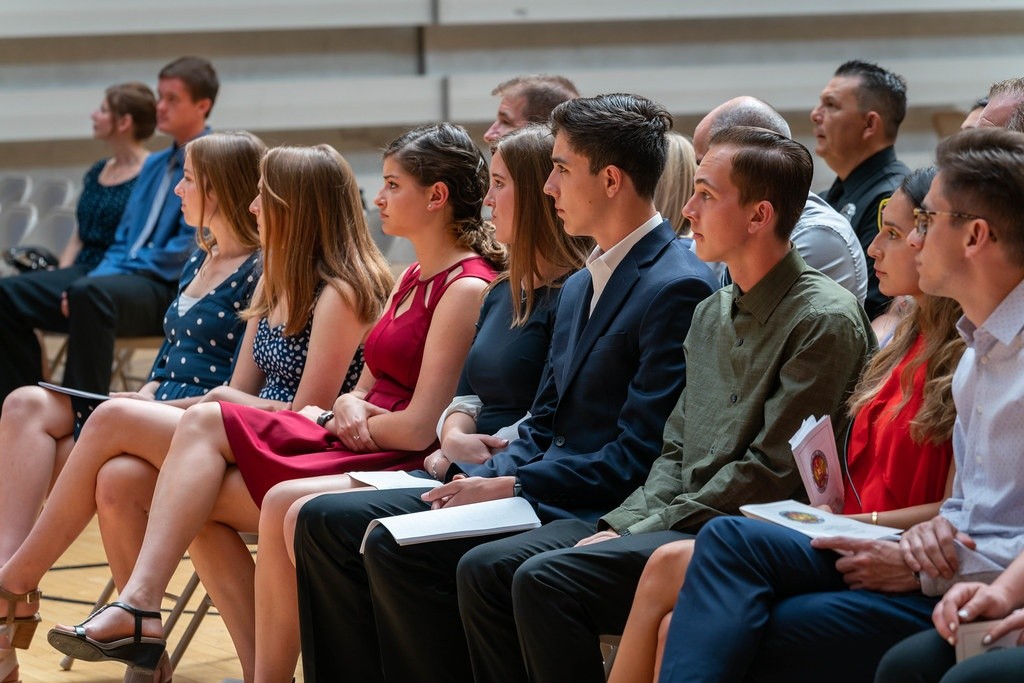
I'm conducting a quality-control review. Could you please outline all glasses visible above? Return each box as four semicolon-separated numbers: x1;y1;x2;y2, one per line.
913;207;998;243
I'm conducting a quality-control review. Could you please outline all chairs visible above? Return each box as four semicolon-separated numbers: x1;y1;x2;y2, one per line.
34;329;256;683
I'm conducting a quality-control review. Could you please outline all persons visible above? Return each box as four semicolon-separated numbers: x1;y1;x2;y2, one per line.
0;56;1024;683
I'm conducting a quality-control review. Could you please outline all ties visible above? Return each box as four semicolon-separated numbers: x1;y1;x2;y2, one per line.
129;154;180;260
826;183;843;205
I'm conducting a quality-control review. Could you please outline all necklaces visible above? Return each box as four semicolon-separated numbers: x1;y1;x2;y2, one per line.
521;286;527;304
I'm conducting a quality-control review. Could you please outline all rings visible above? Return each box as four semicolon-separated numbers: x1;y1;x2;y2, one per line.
353;436;360;442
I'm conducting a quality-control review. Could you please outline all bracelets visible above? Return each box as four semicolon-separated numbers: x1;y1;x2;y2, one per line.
871;511;877;525
513;476;522;497
431;457;442;481
618;529;632;537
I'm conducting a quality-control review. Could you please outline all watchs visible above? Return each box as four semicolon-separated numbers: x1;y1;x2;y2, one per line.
317;411;335;428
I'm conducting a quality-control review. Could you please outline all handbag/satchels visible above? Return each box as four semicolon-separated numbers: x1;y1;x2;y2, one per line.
6;247;58;273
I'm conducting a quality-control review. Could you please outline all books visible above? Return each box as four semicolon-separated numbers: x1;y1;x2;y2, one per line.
739;499;904;558
787;414;844;514
37;382;111;400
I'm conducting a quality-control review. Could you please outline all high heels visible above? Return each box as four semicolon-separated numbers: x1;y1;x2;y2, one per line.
125;650;172;683
0;648;22;683
0;585;41;650
46;602;165;673
